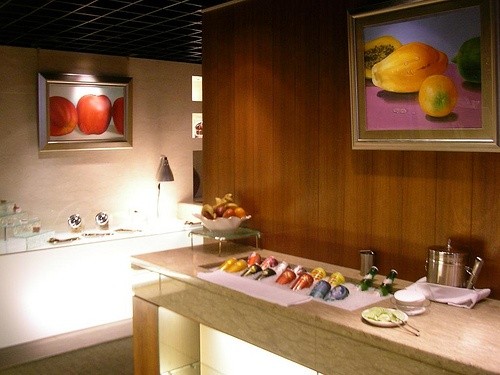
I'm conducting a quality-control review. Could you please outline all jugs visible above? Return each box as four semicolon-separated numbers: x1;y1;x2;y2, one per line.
424;238;484;290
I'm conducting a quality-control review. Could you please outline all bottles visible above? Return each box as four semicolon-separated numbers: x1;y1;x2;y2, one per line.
371;269;398;298
355;265;379;292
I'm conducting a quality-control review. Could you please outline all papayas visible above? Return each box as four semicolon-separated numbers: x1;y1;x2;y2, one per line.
363;36;482;119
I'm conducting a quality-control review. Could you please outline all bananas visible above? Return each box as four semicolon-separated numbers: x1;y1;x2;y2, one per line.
202;204;217;219
216;193;238;208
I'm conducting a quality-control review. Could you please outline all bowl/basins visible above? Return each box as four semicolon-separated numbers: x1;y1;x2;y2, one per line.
192;212;252;234
390;289;425;312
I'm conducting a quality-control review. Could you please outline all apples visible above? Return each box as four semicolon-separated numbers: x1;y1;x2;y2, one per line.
49;94;125;135
203;205;235;219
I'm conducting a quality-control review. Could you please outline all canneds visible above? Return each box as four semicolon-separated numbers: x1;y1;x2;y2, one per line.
216;251;349;303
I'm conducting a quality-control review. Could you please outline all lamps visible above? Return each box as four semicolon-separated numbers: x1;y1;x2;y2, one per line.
154;154;174;218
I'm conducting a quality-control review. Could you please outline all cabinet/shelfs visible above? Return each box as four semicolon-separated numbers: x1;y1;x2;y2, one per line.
0;202;204;369
130;242;500;375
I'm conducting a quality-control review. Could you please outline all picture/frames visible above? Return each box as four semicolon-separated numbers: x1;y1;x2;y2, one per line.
37;71;134;151
347;0;500;151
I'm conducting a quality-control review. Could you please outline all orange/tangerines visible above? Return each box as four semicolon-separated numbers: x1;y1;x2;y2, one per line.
234;207;246;218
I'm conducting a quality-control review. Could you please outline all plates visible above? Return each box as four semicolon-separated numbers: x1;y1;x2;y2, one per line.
361;307;408;328
